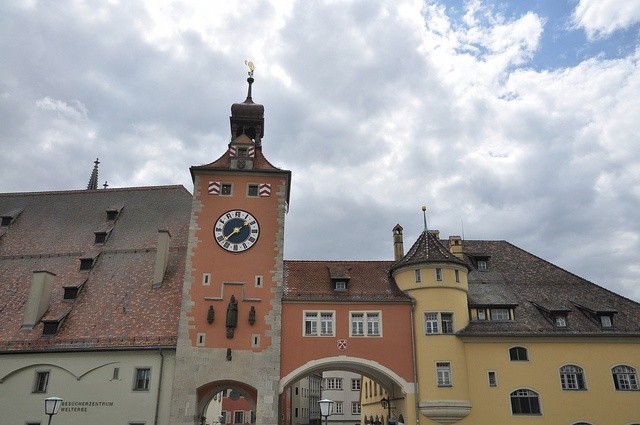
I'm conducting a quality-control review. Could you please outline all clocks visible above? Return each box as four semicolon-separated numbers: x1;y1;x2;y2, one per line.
213;209;261;253
